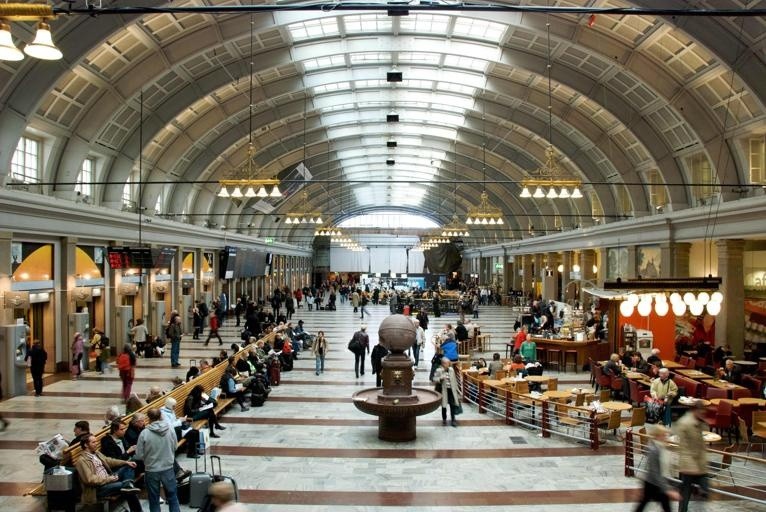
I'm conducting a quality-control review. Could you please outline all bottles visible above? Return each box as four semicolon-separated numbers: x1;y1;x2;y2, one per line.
672;305;707;360
571;388;578;394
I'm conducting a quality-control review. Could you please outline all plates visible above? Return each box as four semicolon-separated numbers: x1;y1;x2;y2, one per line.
534;335;543;338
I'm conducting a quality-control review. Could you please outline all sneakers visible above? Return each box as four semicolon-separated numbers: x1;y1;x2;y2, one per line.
209;423;227;438
120;482;141;493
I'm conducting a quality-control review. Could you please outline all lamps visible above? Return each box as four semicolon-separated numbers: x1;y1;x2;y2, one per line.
216;0;284;200
605;276;725;318
314;140;342;237
518;0;583;200
465;90;505;225
0;0;63;63
285;91;323;224
418;139;470;248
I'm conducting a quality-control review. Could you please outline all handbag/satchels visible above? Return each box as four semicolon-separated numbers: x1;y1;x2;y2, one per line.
454;401;463;415
435;382;443;394
632;401;663;424
348;339;362;355
166;467;191;506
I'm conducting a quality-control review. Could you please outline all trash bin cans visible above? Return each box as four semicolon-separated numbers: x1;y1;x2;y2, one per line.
43;466;77;512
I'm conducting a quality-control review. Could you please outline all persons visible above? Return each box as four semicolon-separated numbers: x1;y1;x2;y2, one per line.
0;373;9;431
40;376;228;511
25;340;47;395
116;277;349;412
71;328;106;378
407;279;605;427
604;341;766;511
349;277;407;387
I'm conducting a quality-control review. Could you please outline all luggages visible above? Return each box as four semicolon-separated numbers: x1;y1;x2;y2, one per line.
250;380;267;408
211;455;240;502
188;442;212;507
271;358;280;386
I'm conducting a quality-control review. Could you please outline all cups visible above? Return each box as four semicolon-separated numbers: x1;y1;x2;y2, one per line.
542;329;561;340
519;372;522;379
615;360;633;373
